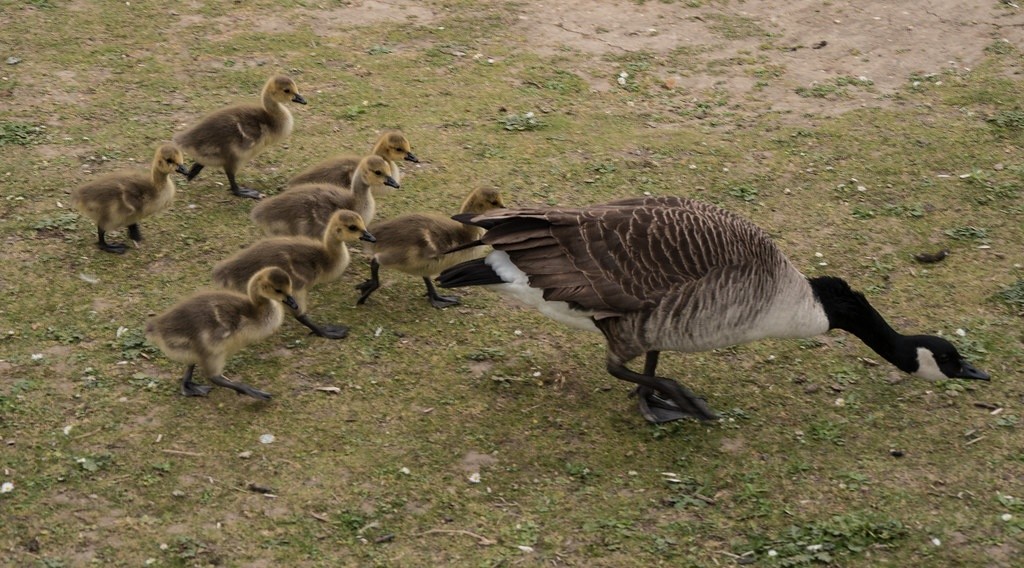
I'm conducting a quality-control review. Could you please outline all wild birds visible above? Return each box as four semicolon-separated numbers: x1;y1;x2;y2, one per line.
277;131;418;197
355;183;506;308
434;198;993;425
173;75;308;200
209;209;375;339
68;145;190;254
248;155;401;245
140;267;299;403
